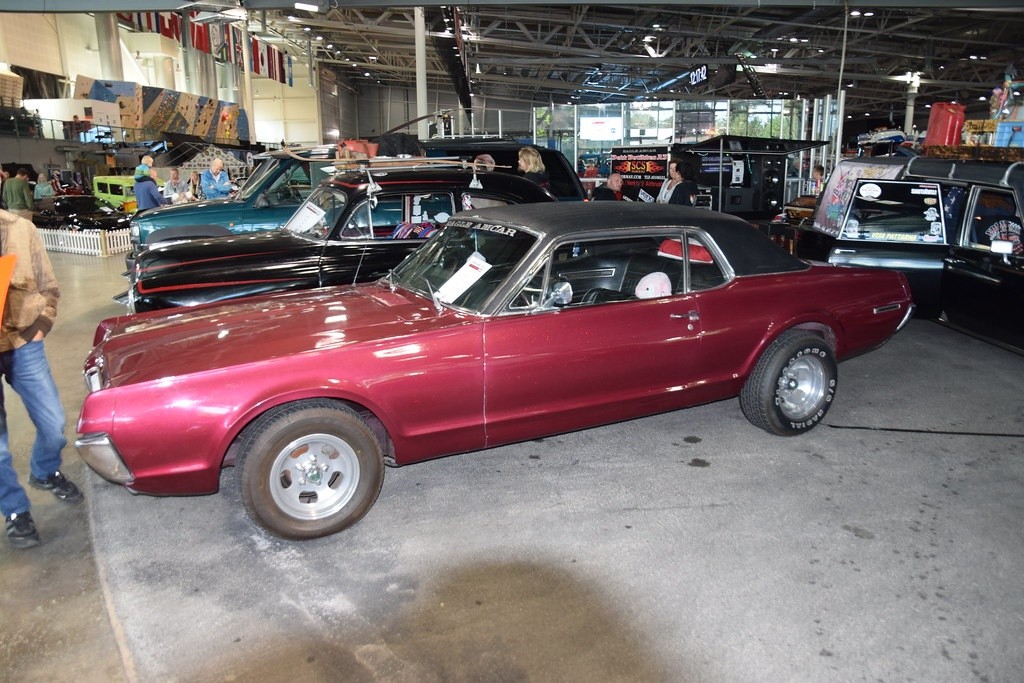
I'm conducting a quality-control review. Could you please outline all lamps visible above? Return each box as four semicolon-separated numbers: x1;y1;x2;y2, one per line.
174;14;181;73
134;12;142;60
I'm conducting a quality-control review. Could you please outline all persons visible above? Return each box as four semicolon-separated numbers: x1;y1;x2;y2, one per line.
656;159;699;206
813;165;824;196
134;169;171;210
0;169;35;221
134;156;156;185
189;171;206;201
51;172;64;195
474;154;495;171
164;169;187;197
202;159;232;199
579;159;610;191
34;173;55;199
518;148;550;190
0;209;83;547
592;173;622;201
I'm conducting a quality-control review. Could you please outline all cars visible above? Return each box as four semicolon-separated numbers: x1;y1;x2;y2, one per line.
31;193;132;232
74;200;918;543
792;154;1024;357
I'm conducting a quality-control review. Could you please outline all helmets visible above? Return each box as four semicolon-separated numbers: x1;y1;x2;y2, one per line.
135;164;151;178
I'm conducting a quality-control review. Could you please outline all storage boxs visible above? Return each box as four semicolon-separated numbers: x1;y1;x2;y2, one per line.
994;122;1024;147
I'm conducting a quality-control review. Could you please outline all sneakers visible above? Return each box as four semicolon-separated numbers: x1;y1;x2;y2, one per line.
6;510;41;547
28;468;83;502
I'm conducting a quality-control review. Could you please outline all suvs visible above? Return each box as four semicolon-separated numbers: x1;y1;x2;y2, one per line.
71;137;593;317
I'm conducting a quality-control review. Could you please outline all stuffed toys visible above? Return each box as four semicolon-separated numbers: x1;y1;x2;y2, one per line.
635;272;672;299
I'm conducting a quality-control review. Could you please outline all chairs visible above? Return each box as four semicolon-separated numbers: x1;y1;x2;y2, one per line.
617;253;725;299
389;222;412;239
970;207;1024;256
418;226;439;238
